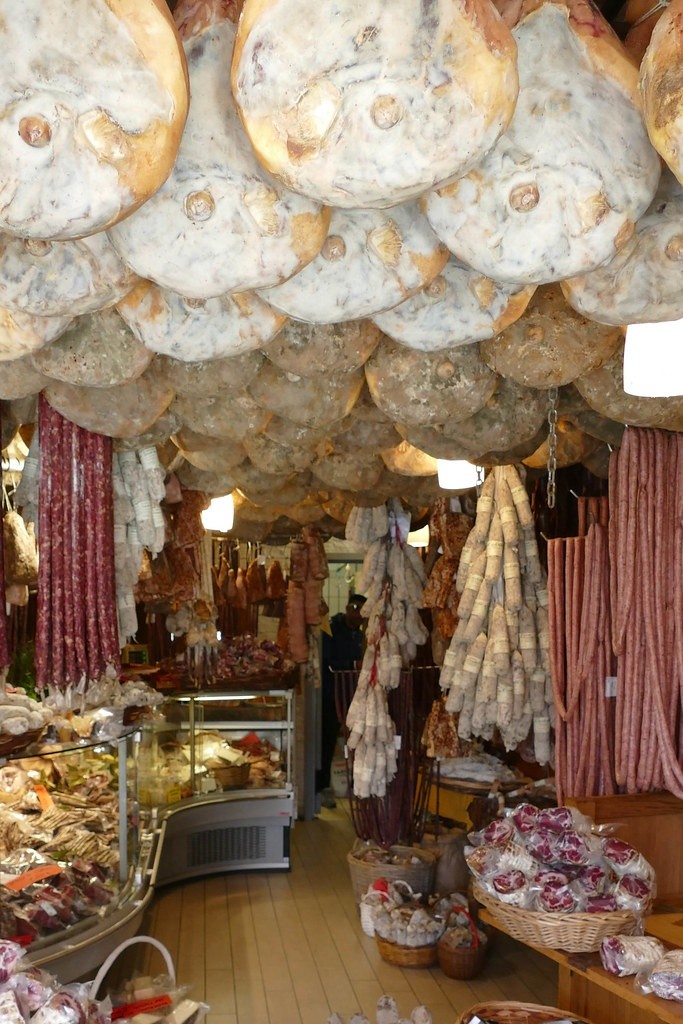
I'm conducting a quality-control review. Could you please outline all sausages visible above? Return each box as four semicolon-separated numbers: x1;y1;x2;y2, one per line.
546;425;683;807
37;390;121;693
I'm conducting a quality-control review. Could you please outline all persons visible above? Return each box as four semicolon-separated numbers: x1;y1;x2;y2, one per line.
317;595;367;809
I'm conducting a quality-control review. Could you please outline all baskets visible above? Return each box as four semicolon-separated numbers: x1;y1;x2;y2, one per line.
455;1000;592;1024
210;761;251;789
434;906;493;981
346;840;436;902
473;883;655;954
368;879;445;968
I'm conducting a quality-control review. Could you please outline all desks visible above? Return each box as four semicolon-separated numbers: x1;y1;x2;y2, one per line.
411;767;493;832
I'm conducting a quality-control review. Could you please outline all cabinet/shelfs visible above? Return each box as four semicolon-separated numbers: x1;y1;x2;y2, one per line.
477;791;683;1024
1;675;300;987
